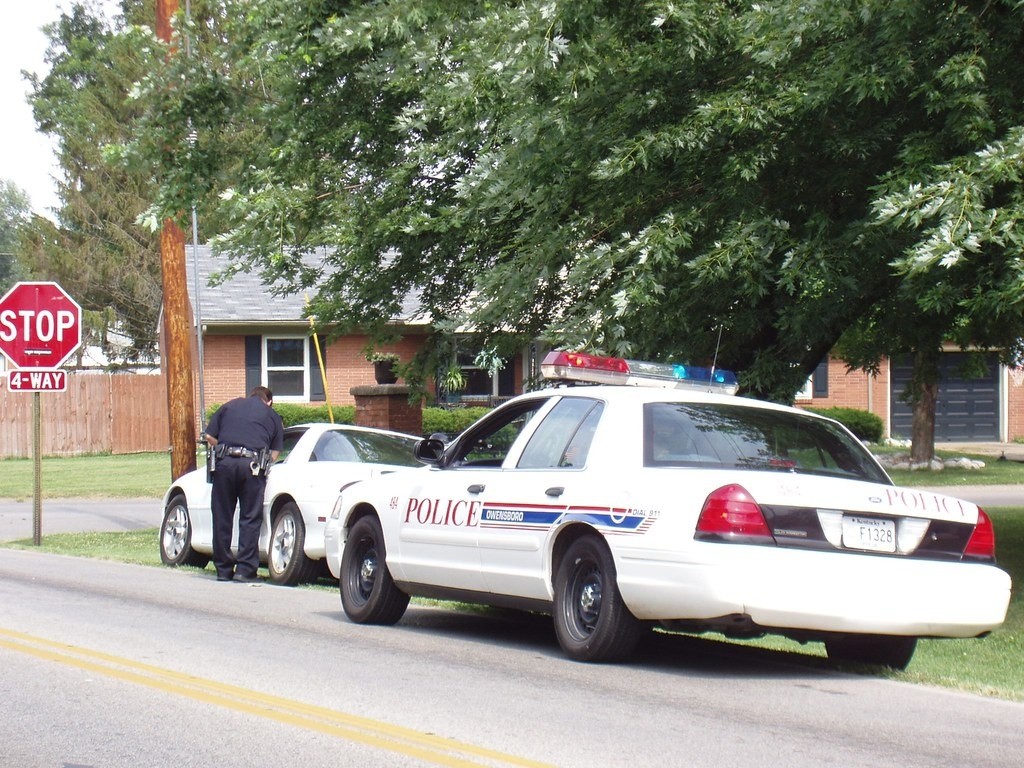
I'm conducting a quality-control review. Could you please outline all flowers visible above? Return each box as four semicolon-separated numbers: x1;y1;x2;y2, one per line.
367;352;400;364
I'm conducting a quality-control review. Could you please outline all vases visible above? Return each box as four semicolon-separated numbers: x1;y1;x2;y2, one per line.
374;360;398;385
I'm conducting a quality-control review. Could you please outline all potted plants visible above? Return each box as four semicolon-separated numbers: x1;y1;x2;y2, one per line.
439;361;467;403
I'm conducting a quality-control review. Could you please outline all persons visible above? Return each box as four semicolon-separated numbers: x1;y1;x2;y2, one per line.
204;386;283;582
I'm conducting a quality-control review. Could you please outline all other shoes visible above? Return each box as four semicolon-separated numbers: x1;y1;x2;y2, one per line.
232;570;257;582
217;570;234;581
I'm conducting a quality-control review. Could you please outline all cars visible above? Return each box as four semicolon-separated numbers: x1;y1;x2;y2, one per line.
158;420;449;587
323;350;1013;677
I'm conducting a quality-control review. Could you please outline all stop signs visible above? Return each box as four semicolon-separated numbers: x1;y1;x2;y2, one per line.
0;281;82;370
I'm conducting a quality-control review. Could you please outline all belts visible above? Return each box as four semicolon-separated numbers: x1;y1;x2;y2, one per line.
225;446;259;457
